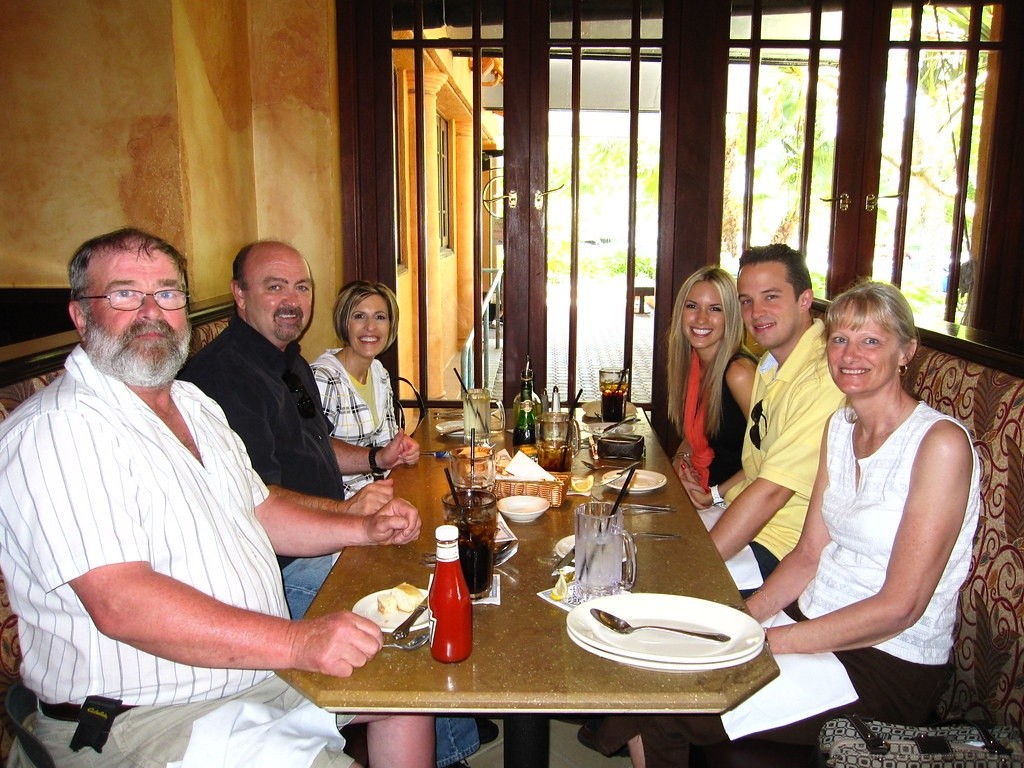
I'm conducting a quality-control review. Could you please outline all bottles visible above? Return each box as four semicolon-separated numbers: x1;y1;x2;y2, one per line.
428;524;474;661
552;386;560;413
513;369;540;445
540;388;549;414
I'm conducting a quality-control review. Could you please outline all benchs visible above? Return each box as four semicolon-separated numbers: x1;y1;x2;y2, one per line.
700;297;1024;768
0;291;236;768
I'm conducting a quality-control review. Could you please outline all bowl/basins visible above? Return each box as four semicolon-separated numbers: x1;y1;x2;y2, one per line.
497;495;550;523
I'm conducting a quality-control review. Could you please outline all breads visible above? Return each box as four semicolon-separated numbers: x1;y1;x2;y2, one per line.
376;594;397;616
495;454;514;476
390;581;423;613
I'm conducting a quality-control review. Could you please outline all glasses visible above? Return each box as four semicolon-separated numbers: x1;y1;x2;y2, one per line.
282;374;315;419
80;290;192;311
749;399;767;450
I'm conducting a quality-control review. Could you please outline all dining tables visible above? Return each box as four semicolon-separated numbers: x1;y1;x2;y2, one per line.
275;407;779;768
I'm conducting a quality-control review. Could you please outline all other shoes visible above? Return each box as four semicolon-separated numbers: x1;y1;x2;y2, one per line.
441;761;471;768
476;719;499;744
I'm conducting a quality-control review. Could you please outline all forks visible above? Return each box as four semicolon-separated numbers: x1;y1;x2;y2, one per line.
428;552;511;565
621;528;682;538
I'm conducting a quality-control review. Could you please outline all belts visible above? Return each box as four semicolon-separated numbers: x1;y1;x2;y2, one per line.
5;681;138;768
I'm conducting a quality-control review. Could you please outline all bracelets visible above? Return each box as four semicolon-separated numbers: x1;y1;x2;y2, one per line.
762;627;770;650
672;451;691;467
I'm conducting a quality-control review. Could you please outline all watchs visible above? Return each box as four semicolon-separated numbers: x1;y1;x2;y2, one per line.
368;447;388;477
708;485;725;506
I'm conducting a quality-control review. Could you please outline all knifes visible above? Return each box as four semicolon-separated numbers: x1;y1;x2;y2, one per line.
589;494;672;510
555;547;575;570
393;594;429;639
594;461;641;487
440;428;465;436
603;416;635;431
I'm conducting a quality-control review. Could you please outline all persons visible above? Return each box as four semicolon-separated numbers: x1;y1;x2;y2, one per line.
0;227;436;768
309;280;501;745
629;283;982;768
173;238;481;768
667;265;759;510
696;243;851;600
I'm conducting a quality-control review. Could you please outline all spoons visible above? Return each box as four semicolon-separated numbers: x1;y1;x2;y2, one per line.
589;608;732;642
382;634;429;649
581;461;626;469
421;539;519;557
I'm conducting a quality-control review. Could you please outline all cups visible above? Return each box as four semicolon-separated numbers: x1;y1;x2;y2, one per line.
441;490;497;598
599;367;629;421
535;412;581;471
574;502;636;597
462;387;506;445
448;446;495;493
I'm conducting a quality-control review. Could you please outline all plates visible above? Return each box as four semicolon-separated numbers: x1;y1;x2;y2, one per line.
566;625;766;674
556;534;637;562
601;469;667;493
352;589;430;634
584;423;635;435
566;593;766;664
436;420;465;438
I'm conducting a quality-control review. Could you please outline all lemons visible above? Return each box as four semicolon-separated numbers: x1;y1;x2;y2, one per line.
551;573;568;600
571;475;594;493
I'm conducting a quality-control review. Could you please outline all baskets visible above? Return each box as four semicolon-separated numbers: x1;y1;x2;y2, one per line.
493;471;571;508
